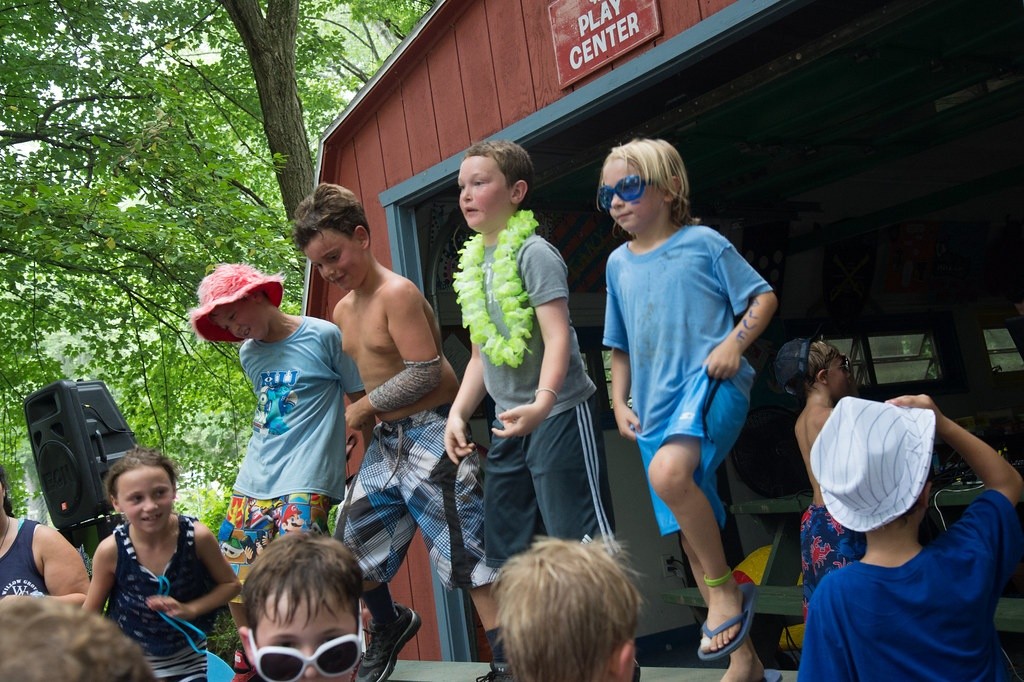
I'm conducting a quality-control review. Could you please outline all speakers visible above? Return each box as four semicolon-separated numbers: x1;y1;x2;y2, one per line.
24;377;140;529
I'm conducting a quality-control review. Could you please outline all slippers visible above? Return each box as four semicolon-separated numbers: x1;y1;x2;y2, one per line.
697;583;759;661
760;669;784;682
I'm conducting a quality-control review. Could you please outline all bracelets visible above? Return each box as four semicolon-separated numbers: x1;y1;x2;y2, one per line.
535;388;559;401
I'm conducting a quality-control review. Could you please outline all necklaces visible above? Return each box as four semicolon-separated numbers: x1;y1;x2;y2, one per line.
452;209;540;369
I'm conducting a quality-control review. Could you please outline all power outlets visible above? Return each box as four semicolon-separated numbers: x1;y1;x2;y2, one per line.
661;554;676;578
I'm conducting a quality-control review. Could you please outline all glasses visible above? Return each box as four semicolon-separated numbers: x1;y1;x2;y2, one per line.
248;598;363;682
597;174;658;209
826;359;851;373
633;658;641;682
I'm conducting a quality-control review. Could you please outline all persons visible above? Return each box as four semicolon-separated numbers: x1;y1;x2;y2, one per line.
79;443;242;681
796;393;1024;682
0;591;159;682
773;325;866;619
238;531;364;682
291;181;509;682
597;137;782;682
489;530;648;682
0;448;92;605
444;138;641;682
188;262;375;682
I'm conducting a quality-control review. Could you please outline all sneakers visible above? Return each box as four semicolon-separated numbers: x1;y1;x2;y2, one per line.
354;602;422;682
475;661;515;682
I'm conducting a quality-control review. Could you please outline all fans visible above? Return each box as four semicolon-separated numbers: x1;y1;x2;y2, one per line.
732;405;811;497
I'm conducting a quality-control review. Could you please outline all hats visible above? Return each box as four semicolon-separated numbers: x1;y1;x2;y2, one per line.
190;263;287;342
810;396;936;532
773;325;822;398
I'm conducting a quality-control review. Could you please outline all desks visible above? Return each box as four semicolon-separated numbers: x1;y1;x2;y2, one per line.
727;483;1024;674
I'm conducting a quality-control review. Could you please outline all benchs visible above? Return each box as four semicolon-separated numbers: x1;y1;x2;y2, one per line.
660;588;1024;635
